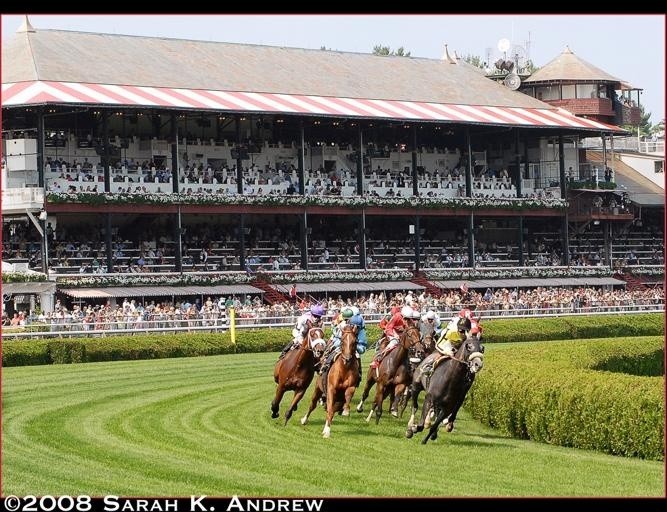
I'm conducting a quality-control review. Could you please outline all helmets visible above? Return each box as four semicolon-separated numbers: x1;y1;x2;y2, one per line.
391;306;435;320
342;306;362;327
457;318;471;331
460;308;472;319
312;306;323;317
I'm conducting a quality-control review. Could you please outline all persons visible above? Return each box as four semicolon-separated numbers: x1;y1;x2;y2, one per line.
0;156;663;375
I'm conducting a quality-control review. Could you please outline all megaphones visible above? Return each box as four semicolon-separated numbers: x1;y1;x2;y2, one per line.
504;74;522;91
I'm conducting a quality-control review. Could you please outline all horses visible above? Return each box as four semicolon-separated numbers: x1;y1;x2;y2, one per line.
270;319;325;418
301;316;484;444
